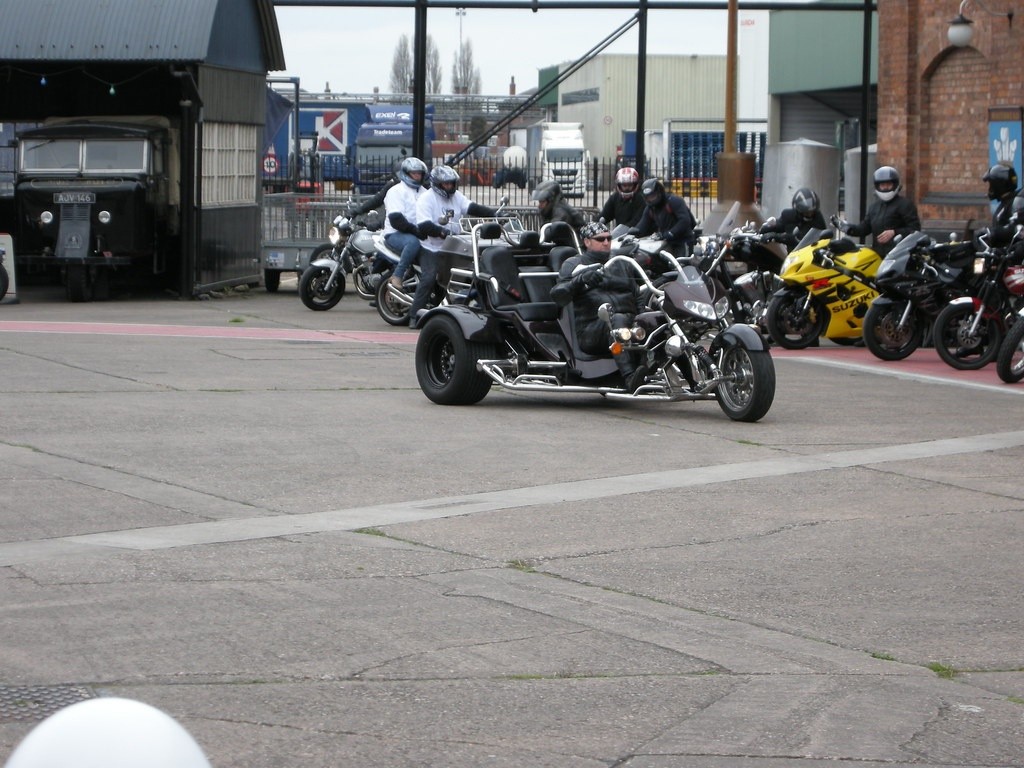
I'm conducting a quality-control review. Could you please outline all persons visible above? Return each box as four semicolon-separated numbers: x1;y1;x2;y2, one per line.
760;188;827;254
551;222;649;394
982;164;1024;269
633;178;694;258
383;158;430;291
593;167;644;237
345;161;401;272
532;180;587;240
409;165;520;329
830;166;921;259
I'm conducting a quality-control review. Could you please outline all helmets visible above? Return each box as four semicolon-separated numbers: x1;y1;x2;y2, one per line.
534;180;562;216
430;165;459;198
792;187;820;222
614;168;640;199
983;163;1018;200
874;166;902;202
399;157;428;189
640;177;666;207
391;158;406;184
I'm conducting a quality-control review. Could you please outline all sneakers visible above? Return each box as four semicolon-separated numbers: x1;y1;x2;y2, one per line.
625;365;647;394
389;272;404;292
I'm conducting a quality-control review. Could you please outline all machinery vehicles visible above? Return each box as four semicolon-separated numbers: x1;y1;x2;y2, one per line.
285;131;325;222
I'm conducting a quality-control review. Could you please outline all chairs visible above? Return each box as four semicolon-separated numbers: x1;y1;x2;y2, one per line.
548;244;578;279
561;255;605;362
479;245;560;321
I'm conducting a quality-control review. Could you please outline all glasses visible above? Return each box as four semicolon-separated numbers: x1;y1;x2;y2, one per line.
589;235;612;242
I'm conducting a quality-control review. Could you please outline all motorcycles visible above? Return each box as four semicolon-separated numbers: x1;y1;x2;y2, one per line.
415;221;776;422
299;197;521;326
931;225;1024;371
863;230;972;361
611;198;788;344
765;226;969;350
996;308;1024;384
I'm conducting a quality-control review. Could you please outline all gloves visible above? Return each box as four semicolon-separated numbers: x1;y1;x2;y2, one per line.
439;227;457;239
412;226;428;240
650;231;665;241
582;270;603;289
344;208;359;220
616;232;632;243
498;209;510;223
830;214;852;235
972;225;987;245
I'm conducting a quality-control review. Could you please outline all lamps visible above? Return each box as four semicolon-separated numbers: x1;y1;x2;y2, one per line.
947;0;1013;49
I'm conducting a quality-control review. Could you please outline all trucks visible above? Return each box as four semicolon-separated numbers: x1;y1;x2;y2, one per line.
527;122;590;199
10;115;181;292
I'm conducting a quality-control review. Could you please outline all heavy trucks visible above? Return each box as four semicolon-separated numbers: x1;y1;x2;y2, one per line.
262;103;435;203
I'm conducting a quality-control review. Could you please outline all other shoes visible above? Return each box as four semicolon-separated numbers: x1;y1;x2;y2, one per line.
410;318;416;330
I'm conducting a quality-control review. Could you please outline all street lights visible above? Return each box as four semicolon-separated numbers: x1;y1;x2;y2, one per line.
455;8;466;144
